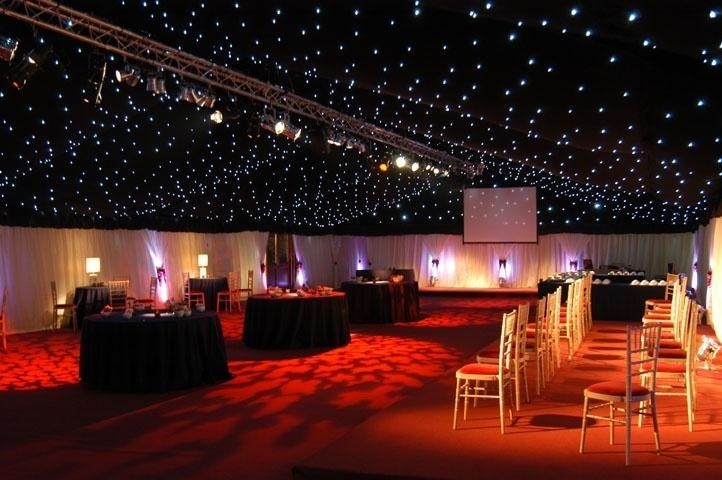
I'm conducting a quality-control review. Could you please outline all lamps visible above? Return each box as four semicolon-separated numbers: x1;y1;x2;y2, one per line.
197;253;209;278
86;257;101;284
0;13;453;184
429;275;439;286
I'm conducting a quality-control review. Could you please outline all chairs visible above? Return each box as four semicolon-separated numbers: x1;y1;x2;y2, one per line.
1;286;12;355
